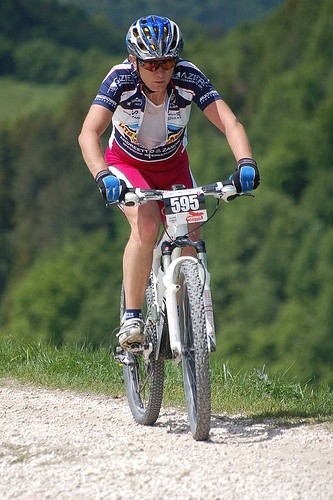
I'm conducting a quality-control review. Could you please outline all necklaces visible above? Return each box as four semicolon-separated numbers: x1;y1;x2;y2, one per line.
146;95;165;114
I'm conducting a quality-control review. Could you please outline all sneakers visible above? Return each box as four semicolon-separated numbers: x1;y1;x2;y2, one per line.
116;317;145;347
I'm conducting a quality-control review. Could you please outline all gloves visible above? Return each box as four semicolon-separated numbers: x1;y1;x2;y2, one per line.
95;169;128;202
228;158;261;193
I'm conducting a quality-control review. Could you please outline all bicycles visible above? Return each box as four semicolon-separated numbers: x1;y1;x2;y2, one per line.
101;169;257;443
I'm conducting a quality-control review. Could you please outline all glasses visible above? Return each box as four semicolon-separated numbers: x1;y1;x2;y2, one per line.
136;58;176;72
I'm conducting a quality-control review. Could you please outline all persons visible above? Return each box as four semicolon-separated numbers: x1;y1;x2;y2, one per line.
77;15;261;347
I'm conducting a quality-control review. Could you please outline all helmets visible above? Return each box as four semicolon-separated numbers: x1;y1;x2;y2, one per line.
125;15;184;62
